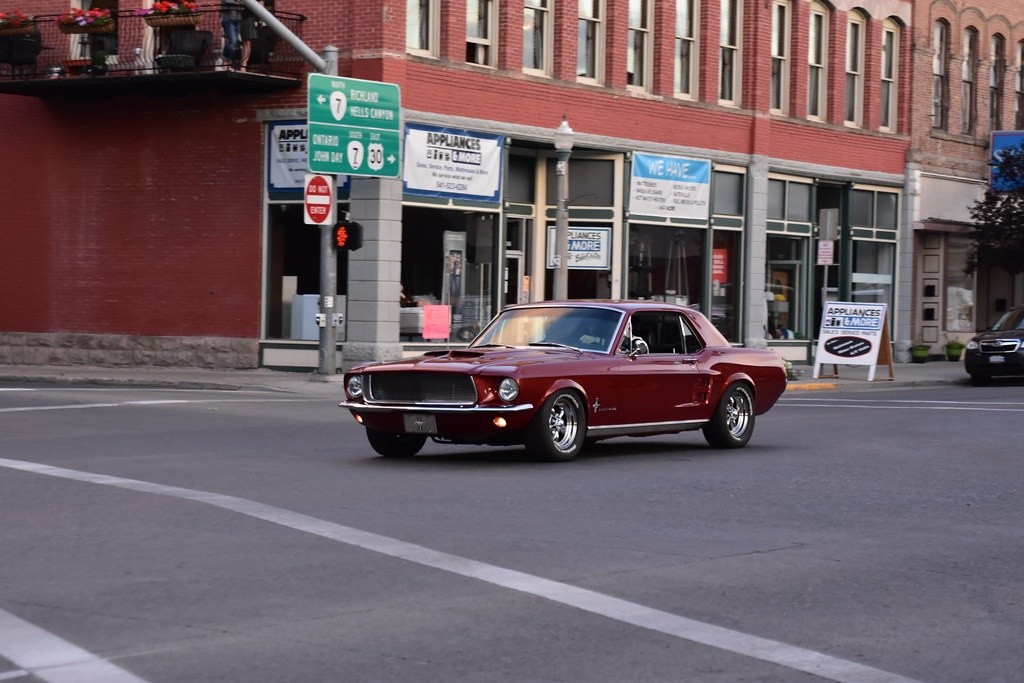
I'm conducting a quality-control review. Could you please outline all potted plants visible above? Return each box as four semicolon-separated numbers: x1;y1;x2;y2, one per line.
911;343;931;363
941;337;966;362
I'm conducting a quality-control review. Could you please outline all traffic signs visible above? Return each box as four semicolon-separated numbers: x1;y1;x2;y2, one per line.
306;73;403;130
306;121;403;181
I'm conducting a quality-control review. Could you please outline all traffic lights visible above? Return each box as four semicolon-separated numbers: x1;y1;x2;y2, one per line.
332;218;355;251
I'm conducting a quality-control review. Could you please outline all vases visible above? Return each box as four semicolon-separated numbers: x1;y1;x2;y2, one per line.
144;12;204;28
0;22;35;36
59;20;116;34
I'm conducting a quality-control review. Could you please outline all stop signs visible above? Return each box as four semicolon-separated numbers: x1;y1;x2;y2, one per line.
306;176;332;224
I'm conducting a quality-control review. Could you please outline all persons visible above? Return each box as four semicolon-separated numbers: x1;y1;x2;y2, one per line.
218;0;265;72
584;324;649;355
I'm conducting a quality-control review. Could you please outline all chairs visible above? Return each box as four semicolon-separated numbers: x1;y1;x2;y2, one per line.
155;30;213;74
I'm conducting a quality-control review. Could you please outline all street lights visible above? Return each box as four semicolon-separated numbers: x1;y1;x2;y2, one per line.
551;112;576;300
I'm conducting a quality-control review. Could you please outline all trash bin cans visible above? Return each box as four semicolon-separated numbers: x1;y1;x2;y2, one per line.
808;340;818;366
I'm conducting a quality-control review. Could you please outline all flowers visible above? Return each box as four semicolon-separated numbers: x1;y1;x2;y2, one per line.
0;11;29;27
135;0;196;17
55;6;111;29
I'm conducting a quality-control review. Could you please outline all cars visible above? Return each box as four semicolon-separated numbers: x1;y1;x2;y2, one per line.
963;306;1024;387
401;287;738;355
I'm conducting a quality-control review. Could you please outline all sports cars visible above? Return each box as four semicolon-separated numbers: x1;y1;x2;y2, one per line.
338;297;789;464
